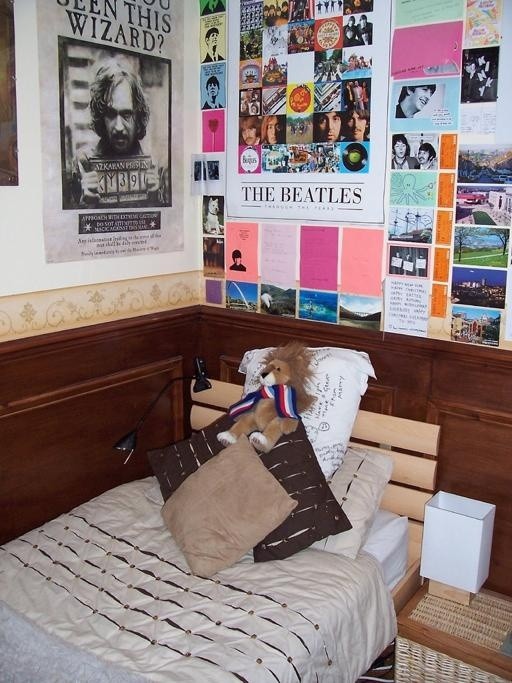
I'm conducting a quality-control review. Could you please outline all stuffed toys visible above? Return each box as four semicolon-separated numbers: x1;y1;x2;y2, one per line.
216;339;315;455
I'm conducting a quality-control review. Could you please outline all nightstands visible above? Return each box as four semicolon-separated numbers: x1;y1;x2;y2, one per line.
396;580;512;683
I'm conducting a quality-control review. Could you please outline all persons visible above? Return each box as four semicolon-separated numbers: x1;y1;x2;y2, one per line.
418;142;437;170
392;251;428;276
65;64;171;209
461;54;497;103
202;76;224;110
229;250;246;271
391;134;420;169
201;27;225;63
396;85;437;118
240;0;373;146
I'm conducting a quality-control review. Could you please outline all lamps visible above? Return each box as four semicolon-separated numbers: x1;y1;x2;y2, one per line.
112;356;212;453
420;491;495;607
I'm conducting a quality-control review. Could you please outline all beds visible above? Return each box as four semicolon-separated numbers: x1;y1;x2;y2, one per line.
1;377;441;683
